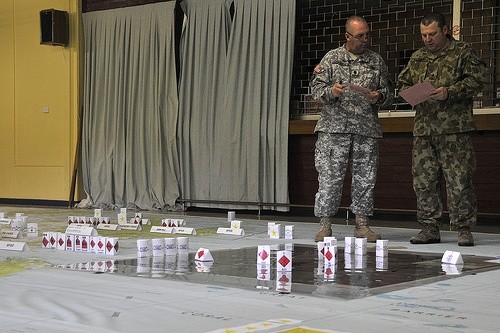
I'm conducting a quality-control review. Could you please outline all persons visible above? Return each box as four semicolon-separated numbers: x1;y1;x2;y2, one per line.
308;15;394;245
397;11;486;246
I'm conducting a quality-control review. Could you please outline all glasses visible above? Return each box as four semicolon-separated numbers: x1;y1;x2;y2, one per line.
346;31;370;41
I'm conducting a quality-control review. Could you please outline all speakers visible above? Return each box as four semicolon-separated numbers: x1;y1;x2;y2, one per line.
39;8;69;46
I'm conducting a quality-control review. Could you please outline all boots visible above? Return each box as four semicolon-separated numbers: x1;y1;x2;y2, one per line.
354;218;381;243
315;219;332;242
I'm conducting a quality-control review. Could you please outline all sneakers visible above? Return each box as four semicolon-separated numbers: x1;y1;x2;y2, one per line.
410;225;441;244
457;226;474;246
